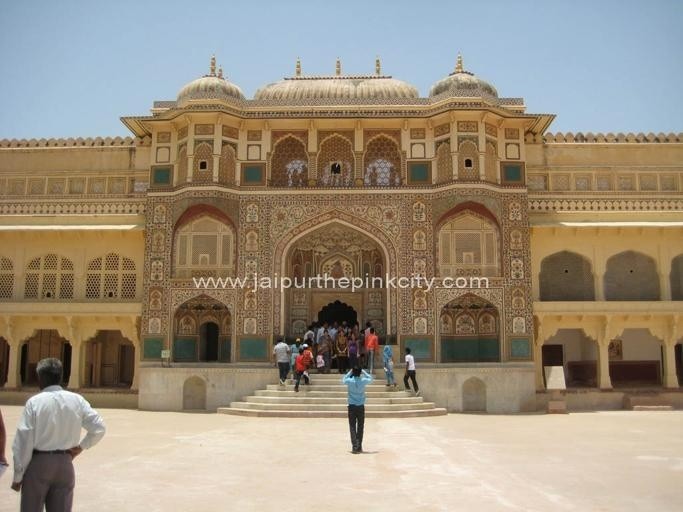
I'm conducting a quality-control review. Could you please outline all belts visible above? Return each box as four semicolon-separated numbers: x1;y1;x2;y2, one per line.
32;449;71;455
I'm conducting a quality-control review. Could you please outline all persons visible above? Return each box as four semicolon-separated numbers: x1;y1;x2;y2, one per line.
9;357;105;512
271;315;398;392
0;408;12;470
401;346;422;397
342;364;372;453
